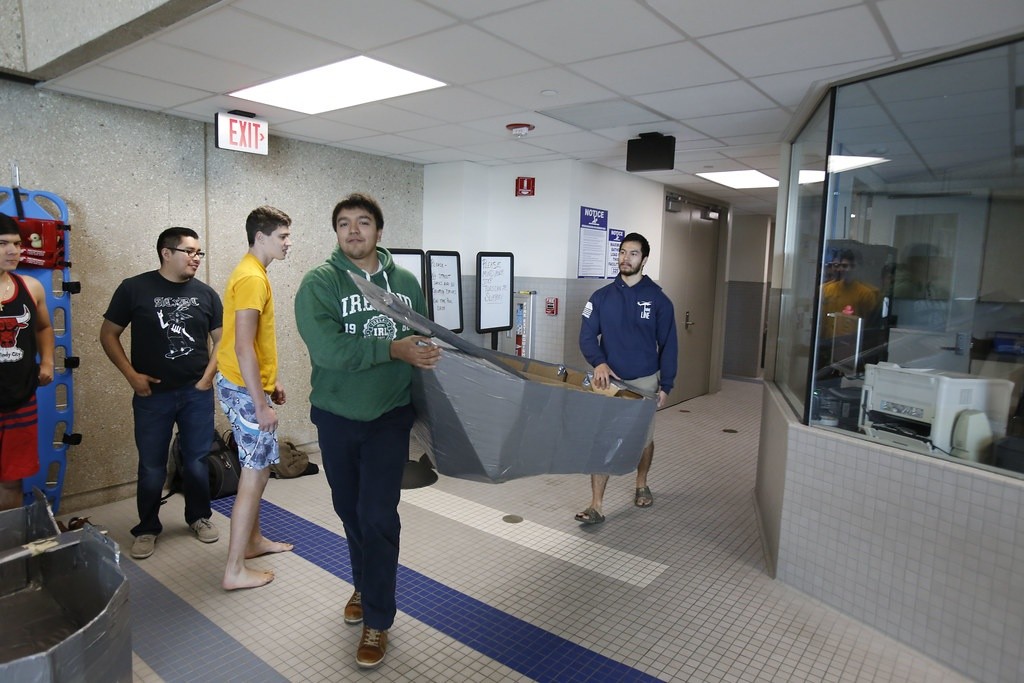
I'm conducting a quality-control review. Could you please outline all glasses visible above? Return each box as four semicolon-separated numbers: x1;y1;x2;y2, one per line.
167;246;204;258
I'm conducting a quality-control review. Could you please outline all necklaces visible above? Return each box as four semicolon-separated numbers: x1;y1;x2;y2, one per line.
0;275;11;302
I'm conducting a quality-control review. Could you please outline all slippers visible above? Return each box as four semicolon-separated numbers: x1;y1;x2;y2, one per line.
575;508;604;524
635;486;653;507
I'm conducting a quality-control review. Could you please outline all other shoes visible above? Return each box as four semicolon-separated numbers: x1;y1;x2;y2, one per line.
68;516;105;534
58;521;67;533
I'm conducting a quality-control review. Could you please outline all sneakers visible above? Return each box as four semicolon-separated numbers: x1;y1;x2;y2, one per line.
344;591;363;625
190;518;219;542
130;535;157;558
356;625;387;668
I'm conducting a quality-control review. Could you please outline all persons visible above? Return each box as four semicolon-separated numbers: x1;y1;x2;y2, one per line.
817;243;965;347
294;194;440;668
215;206;293;590
98;225;225;558
574;232;679;525
0;213;54;514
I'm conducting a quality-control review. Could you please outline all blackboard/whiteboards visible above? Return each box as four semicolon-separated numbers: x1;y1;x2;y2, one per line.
427;250;465;334
387;248;426;298
476;252;514;334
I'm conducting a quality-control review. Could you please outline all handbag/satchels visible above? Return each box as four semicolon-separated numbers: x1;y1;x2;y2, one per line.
270;444;308;477
223;429;238;451
176;453;240;499
173;429;227;455
